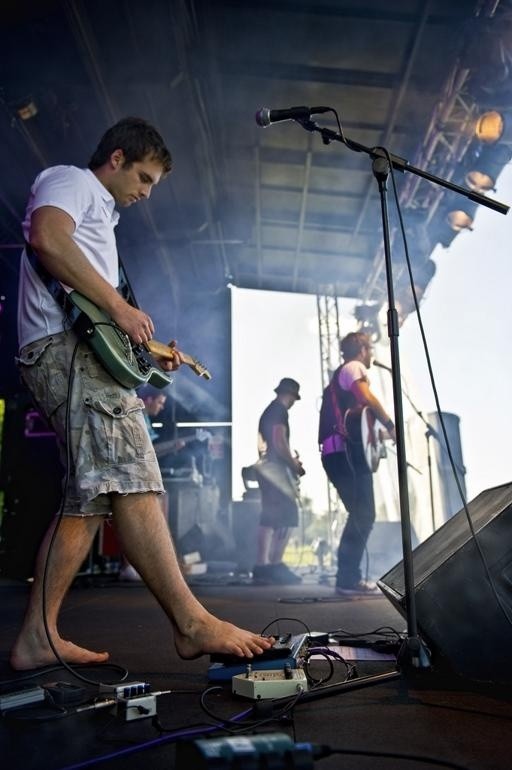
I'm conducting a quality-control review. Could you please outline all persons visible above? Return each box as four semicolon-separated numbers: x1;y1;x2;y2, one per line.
137;384;185;459
9;117;277;672
253;378;305;584
318;332;396;595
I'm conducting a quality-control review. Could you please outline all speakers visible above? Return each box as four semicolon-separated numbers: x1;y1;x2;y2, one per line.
376;482;512;678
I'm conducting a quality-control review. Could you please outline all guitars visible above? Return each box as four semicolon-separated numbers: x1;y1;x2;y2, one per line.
287;449;301;497
153;428;212;458
342;404;391;475
69;290;212;389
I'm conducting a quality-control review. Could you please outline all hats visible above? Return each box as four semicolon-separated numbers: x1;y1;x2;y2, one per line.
273;377;302;400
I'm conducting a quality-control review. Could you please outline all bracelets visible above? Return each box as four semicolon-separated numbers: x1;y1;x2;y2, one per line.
384;419;394;433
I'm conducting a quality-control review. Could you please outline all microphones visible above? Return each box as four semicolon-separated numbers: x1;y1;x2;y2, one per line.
373;360;392;372
255;106;332;128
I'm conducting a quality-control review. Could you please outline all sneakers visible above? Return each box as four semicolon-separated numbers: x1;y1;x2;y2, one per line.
334;578;378;596
251;563;302;586
117;565;143;583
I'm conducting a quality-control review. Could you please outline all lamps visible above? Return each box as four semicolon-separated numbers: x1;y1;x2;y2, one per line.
443;101;512;230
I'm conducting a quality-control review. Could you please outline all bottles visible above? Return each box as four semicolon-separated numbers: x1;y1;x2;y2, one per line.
192;733;320;759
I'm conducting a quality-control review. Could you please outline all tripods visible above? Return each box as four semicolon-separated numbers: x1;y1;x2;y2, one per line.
250;128;512;719
